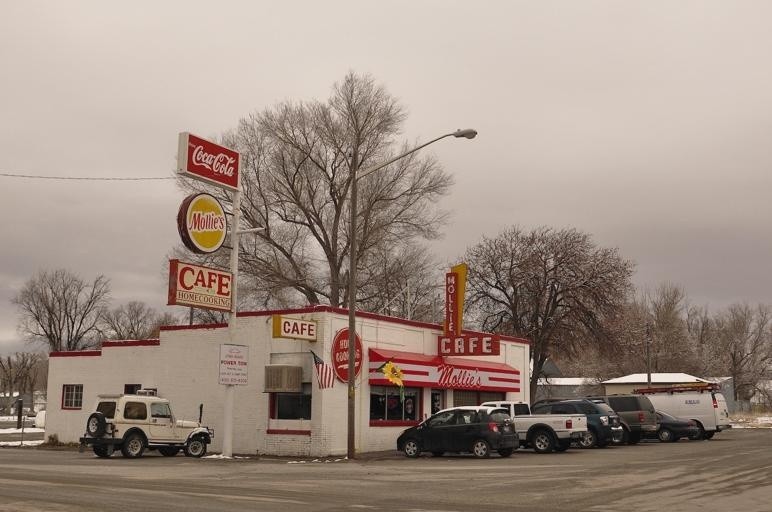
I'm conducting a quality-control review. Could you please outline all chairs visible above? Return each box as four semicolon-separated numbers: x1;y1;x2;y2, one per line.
457;414;465;425
470;415;481;423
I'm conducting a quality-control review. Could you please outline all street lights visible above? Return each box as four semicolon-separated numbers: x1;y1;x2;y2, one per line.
343;124;482;460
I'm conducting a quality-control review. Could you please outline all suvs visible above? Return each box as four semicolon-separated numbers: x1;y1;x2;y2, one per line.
79;388;214;460
